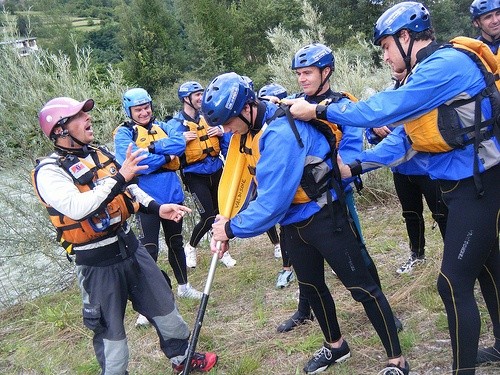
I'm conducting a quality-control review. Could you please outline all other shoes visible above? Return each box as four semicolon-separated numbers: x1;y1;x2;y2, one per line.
474;346;500;366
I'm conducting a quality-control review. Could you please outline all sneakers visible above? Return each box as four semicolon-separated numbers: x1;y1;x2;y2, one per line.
184;241;198;269
135;314;152;330
171;352;218;375
277;310;314;333
274;243;283;258
396;255;427;276
276;268;295;290
218;251;237;269
303;338;351;375
384;361;410;375
177;282;204;300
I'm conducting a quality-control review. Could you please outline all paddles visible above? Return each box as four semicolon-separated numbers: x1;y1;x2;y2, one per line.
182;133;256;375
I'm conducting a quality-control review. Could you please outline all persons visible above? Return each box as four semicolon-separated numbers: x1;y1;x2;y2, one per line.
206;76;282;258
165;81;236;269
261;0;500;375
33;95;218;375
202;70;410;375
259;44;364;287
113;88;203;329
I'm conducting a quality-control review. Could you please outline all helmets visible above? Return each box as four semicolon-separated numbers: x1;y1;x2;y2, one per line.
292;43;335;71
177;81;205;104
373;1;431;46
38;97;95;142
256;83;287;102
469;0;500;20
121;88;154;118
200;71;255;127
